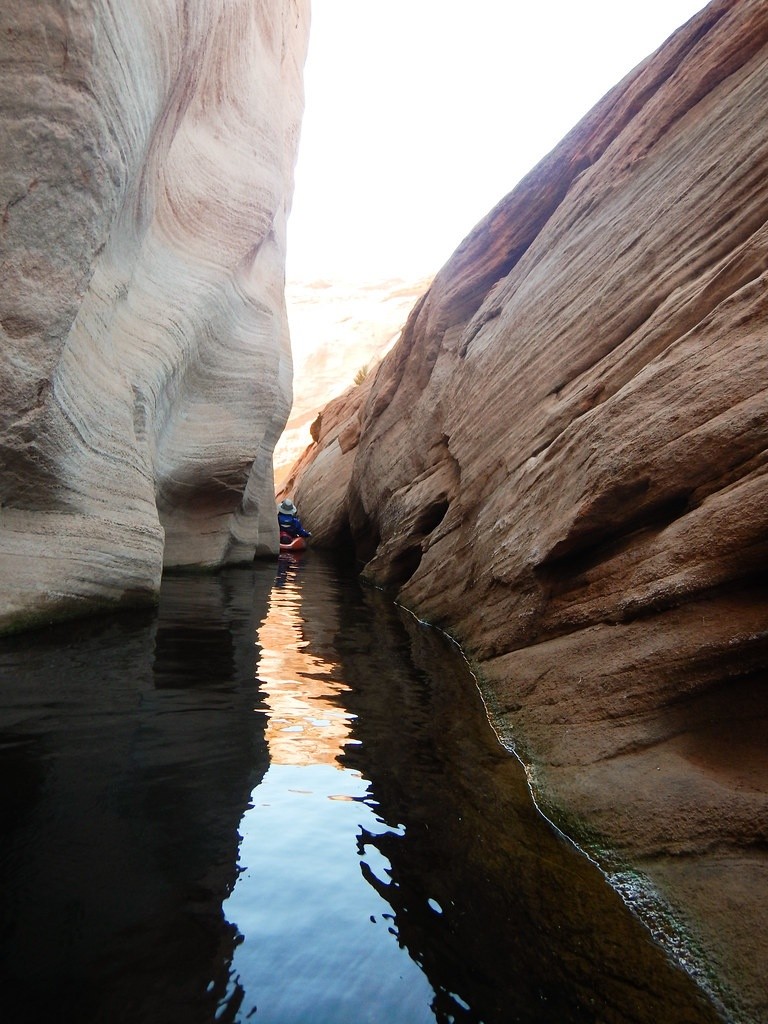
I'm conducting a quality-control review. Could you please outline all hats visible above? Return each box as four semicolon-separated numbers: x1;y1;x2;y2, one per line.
277;499;297;514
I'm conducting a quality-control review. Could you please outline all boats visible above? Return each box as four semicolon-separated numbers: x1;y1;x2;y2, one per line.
279;530;308;551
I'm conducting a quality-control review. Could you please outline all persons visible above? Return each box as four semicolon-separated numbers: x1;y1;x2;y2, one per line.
277;499;313;539
